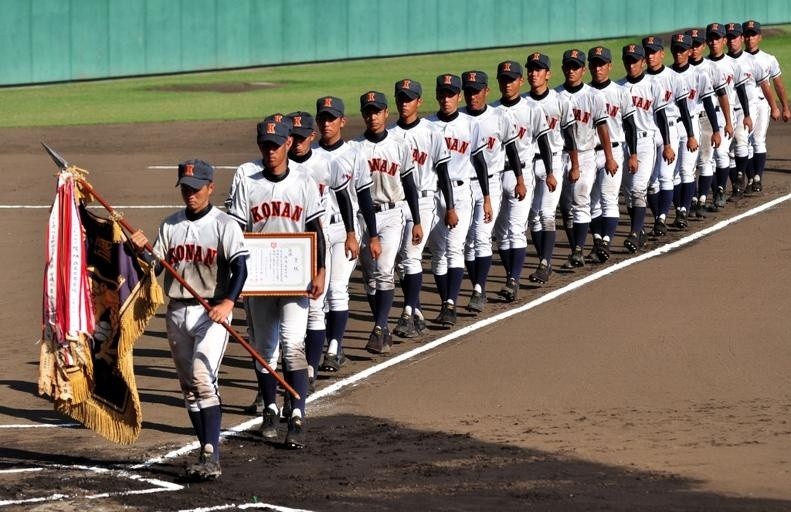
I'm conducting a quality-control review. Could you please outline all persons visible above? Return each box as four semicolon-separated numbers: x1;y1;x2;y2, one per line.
353;92;422;354
522;51;579;283
683;29;733;218
227;115;318;417
641;36;699;236
289;111;359;398
459;71;526;312
491;59;557;301
311;97;381;370
387;78;457;338
725;23;780;199
705;22;753;206
227;120;326;447
615;43;676;255
553;49;618;268
667;35;720;229
743;20;789;189
424;75;493;329
588;46;638;264
131;161;248;482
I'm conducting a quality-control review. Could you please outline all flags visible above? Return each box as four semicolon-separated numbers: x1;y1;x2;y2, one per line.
36;173;165;446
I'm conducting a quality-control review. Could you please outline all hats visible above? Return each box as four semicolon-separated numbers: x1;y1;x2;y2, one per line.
589;46;613;63
285;111;316;137
436;74;462;95
497;61;524;78
257;122;290;146
725;23;744;36
623;44;645;60
642;36;665;51
563;49;587;67
671;36;694;49
743;21;762;34
707;23;727;38
316;96;345;116
395;79;423;100
462;71;490;89
686;29;708;44
525;53;552;70
264;112;295;133
176;159;214;190
360;91;388;109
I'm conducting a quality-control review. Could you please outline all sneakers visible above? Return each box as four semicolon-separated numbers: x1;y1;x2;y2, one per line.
468;291;487;313
285;416;306;449
382;329;393;352
437;302;447;323
696;202;707;218
259;408;282;437
394;314;415;336
441;303;458;326
674;211;690;228
647;219;668;238
585;240;598;265
754;178;763;192
728;185;744;202
187;455;222;478
690;200;700;218
710;196;720;211
306;377;315;396
590;240;611;261
319;350;347;371
501;280;520;301
282;398;293;418
561;249;585;270
409;319;426;338
366;327;384;352
246;394;266;413
746;180;754;194
625;234;641;253
639;229;646;248
716;191;726;207
530;264;552;284
